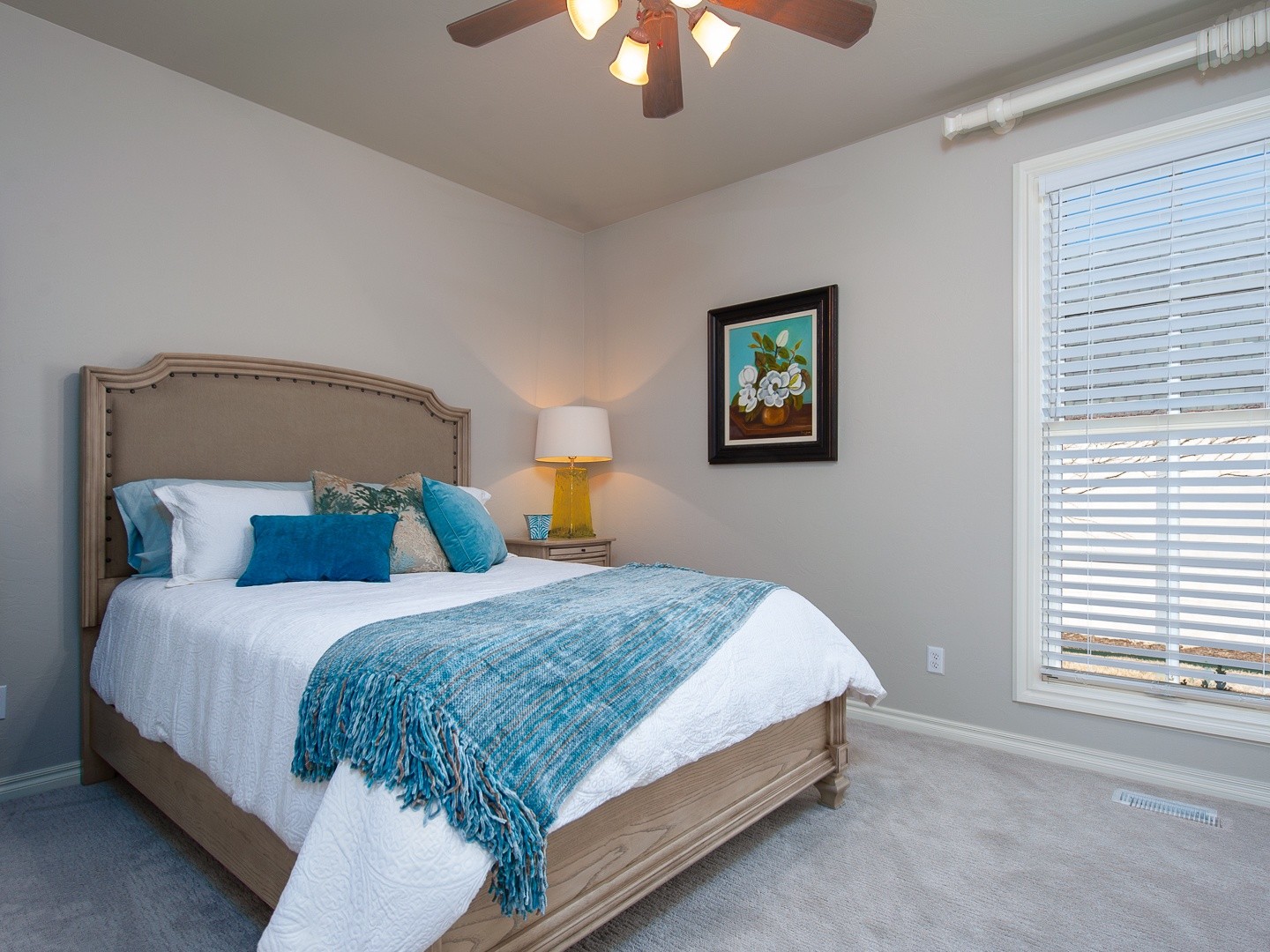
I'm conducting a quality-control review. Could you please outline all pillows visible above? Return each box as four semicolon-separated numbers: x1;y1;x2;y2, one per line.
309;467;508;575
236;512;399;588
153;483;312;589
112;472;313;578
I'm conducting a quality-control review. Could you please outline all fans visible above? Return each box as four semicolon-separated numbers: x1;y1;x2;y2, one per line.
448;0;879;120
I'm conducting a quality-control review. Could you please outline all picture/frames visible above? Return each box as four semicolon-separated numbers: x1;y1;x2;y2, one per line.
706;285;838;466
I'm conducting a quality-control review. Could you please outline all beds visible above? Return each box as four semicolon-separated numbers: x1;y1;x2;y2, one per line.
75;351;890;952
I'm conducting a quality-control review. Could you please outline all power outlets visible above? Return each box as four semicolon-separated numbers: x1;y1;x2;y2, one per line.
928;646;944;675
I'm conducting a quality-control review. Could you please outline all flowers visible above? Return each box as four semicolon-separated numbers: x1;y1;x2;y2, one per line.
731;329;812;426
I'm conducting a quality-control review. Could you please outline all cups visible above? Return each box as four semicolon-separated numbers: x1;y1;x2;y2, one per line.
524;514;553;540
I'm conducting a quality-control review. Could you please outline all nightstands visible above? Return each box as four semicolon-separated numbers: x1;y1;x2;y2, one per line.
503;536;617;568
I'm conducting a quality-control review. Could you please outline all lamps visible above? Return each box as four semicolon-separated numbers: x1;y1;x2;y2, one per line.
566;0;741;86
534;404;613;537
